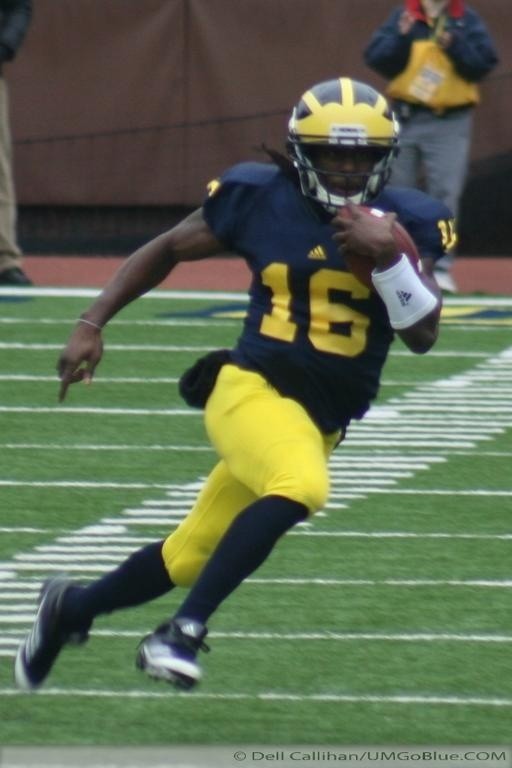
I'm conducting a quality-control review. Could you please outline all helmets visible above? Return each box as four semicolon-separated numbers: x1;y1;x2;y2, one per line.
286;76;402;150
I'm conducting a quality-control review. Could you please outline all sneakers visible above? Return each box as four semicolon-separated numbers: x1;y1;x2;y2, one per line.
14;573;88;693
1;267;34;287
433;270;459;296
133;622;204;690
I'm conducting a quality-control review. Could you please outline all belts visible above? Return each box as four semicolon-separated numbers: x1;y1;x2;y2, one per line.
394;98;474;118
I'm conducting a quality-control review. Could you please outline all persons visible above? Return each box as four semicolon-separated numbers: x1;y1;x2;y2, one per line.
360;0;503;299
0;0;35;287
12;74;461;696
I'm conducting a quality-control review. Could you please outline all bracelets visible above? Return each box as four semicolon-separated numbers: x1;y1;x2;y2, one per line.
76;317;104;332
370;251;440;331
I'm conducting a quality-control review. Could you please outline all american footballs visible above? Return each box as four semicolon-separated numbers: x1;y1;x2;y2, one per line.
337;206;423;291
